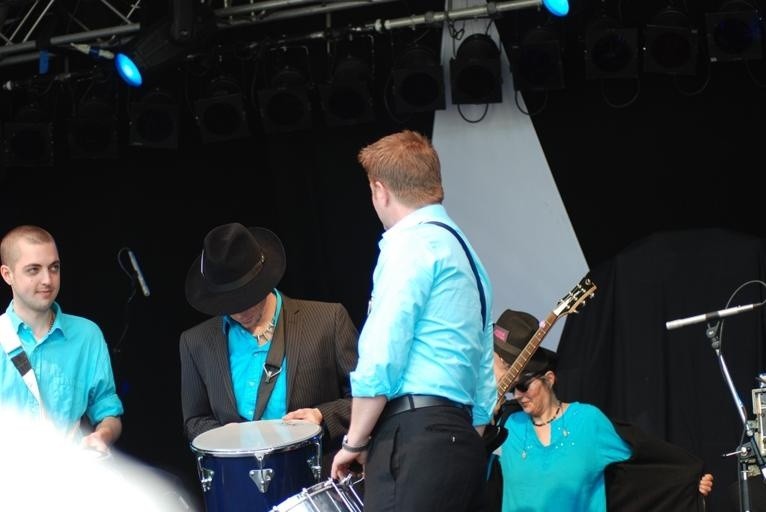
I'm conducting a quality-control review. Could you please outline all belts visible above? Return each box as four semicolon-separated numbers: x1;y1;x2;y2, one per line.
378;394;460;418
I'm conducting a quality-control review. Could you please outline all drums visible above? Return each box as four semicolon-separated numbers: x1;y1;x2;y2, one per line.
265;472;364;512
191;418;325;511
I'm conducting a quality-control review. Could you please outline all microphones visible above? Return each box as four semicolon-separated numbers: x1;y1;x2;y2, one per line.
125;247;154;298
662;302;757;334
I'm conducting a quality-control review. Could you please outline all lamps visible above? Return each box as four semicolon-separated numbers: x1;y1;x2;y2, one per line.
257;56;317;142
583;17;645;87
541;0;582;18
644;11;700;76
1;95;61;171
318;48;378;132
61;86;126;171
114;0;216;87
509;28;568;92
389;42;447;116
449;33;504;106
128;73;186;153
704;2;766;65
193;62;250;146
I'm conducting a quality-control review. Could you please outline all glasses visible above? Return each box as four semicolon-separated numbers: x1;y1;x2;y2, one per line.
507;374;542;392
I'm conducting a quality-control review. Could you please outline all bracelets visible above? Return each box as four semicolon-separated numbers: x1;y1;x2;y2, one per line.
341;433;372;453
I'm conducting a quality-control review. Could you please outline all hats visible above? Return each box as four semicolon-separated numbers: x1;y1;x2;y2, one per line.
520;347;563;375
184;223;286;316
493;309;544;371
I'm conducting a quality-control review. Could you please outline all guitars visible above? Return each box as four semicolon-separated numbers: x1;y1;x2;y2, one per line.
481;277;598;458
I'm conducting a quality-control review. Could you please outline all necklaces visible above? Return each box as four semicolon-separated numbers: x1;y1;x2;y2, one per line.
531;398;563;426
252;305;278;342
45;310;54;336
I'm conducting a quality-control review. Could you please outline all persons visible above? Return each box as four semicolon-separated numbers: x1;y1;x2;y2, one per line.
0;224;124;452
177;223;360;483
329;129;499;512
493;309;548;405
496;346;715;511
